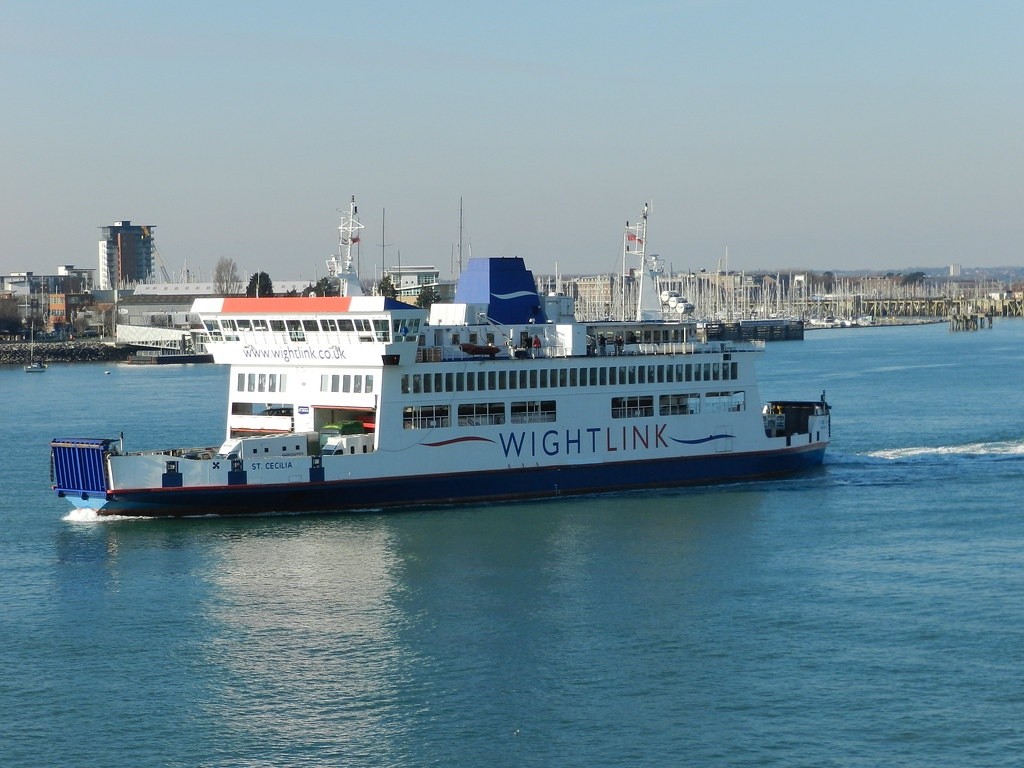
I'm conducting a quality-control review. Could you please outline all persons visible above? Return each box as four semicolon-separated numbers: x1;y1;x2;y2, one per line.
631;333;636;344
587;335;607;354
289;324;306;341
70;333;73;340
208;324;217;335
396;321;409;342
506;334;541;359
737;402;740;411
615;335;624;352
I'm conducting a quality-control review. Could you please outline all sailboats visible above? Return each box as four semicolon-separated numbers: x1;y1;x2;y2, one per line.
23;320;48;373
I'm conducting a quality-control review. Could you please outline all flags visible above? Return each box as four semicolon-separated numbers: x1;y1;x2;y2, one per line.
628;231;642;244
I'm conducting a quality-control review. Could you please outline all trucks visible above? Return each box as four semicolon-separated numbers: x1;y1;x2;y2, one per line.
319;432;376;456
318;420;365;452
212;432;308;461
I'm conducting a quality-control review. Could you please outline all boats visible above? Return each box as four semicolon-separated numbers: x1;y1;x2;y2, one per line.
49;196;833;520
